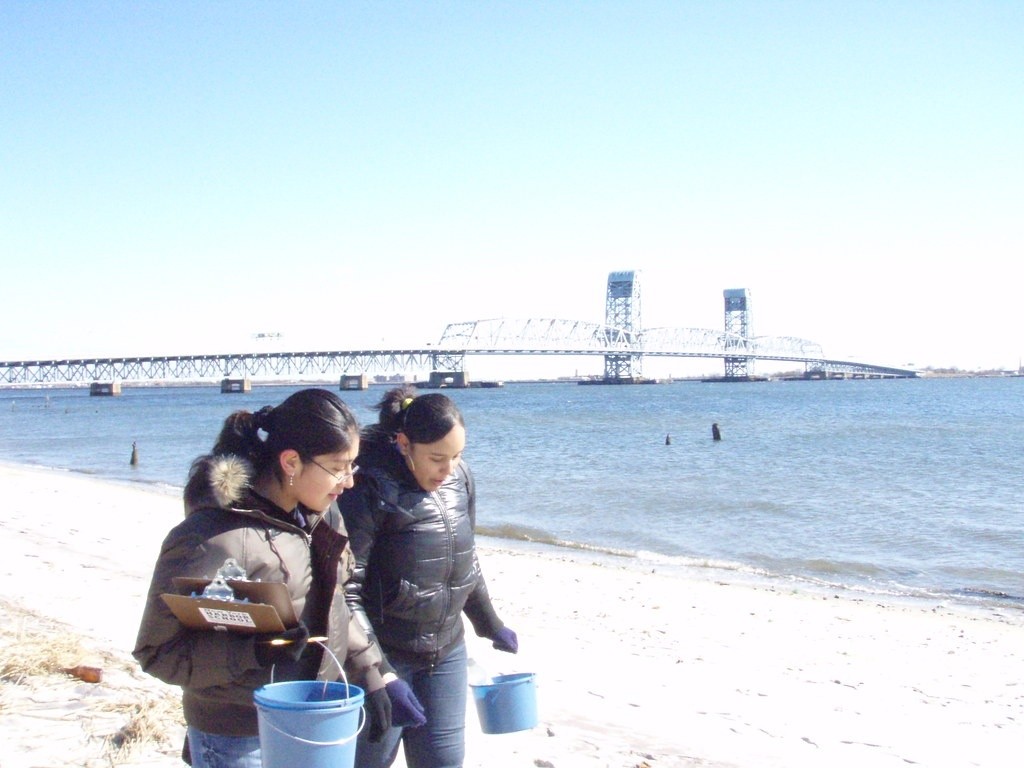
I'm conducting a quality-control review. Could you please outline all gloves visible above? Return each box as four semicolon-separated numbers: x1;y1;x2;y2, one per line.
256;620;310;668
364;686;393;743
386;679;427;727
490;626;518;654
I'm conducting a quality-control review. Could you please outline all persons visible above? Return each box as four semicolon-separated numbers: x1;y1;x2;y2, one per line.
131;388;393;768
336;387;518;768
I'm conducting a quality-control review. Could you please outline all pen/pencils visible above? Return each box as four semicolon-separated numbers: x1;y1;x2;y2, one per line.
267;636;330;645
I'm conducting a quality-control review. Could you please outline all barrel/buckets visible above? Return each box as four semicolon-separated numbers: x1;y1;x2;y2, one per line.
468;672;538;734
253;641;366;768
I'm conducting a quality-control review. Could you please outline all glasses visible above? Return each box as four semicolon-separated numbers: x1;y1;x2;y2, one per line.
308;456;360;485
